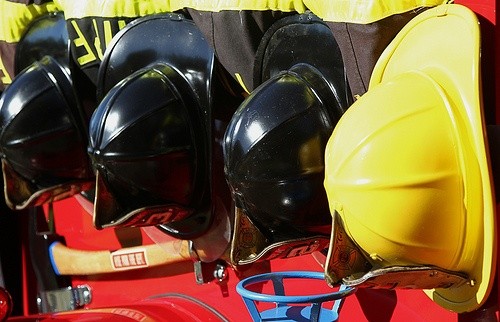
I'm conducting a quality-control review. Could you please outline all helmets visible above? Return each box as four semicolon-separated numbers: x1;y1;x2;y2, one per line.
323;4;497;313
0;12;94;213
223;16;350;269
87;13;215;239
